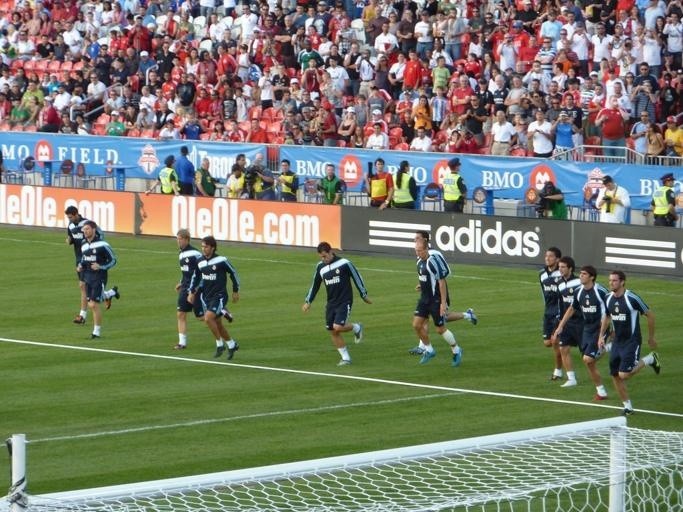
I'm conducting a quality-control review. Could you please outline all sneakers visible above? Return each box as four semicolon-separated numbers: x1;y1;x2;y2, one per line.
173;344;187;350
649;349;663;376
465;306;478;326
212;341;239;361
103;284;120;310
449;346;464;368
335;358;353;367
221;307;233;323
407;345;437;366
591;393;609;402
88;334;100;340
617;408;635;417
547;374;578;388
352;322;363;345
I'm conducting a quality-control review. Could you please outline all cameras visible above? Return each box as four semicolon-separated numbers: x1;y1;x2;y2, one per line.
645;26;652;33
667;16;675;25
639;85;646;91
602;196;612;213
560;114;568;120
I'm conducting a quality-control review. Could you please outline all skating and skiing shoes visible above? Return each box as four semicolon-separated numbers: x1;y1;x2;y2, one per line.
71;315;86;325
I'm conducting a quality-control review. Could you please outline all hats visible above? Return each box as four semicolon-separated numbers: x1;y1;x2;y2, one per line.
163;155;176;164
446;157;462;168
0;0;683;138
659;172;676;182
601;175;613;185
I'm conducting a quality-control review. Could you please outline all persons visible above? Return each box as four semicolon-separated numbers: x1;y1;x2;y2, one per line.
555;255;585;388
363;158;418;210
317;166;344;204
184;235;240;361
145;146;216;196
301;242;372;368
538;247;565;381
0;1;682;165
597;270;662;417
538;181;568;219
407;230;477;355
411;239;463;368
75;221;120;342
64;206;112;325
170;229;233;350
225;153;299;202
651;172;678;226
596;175;631;223
550;264;616;402
442;158;467;213
